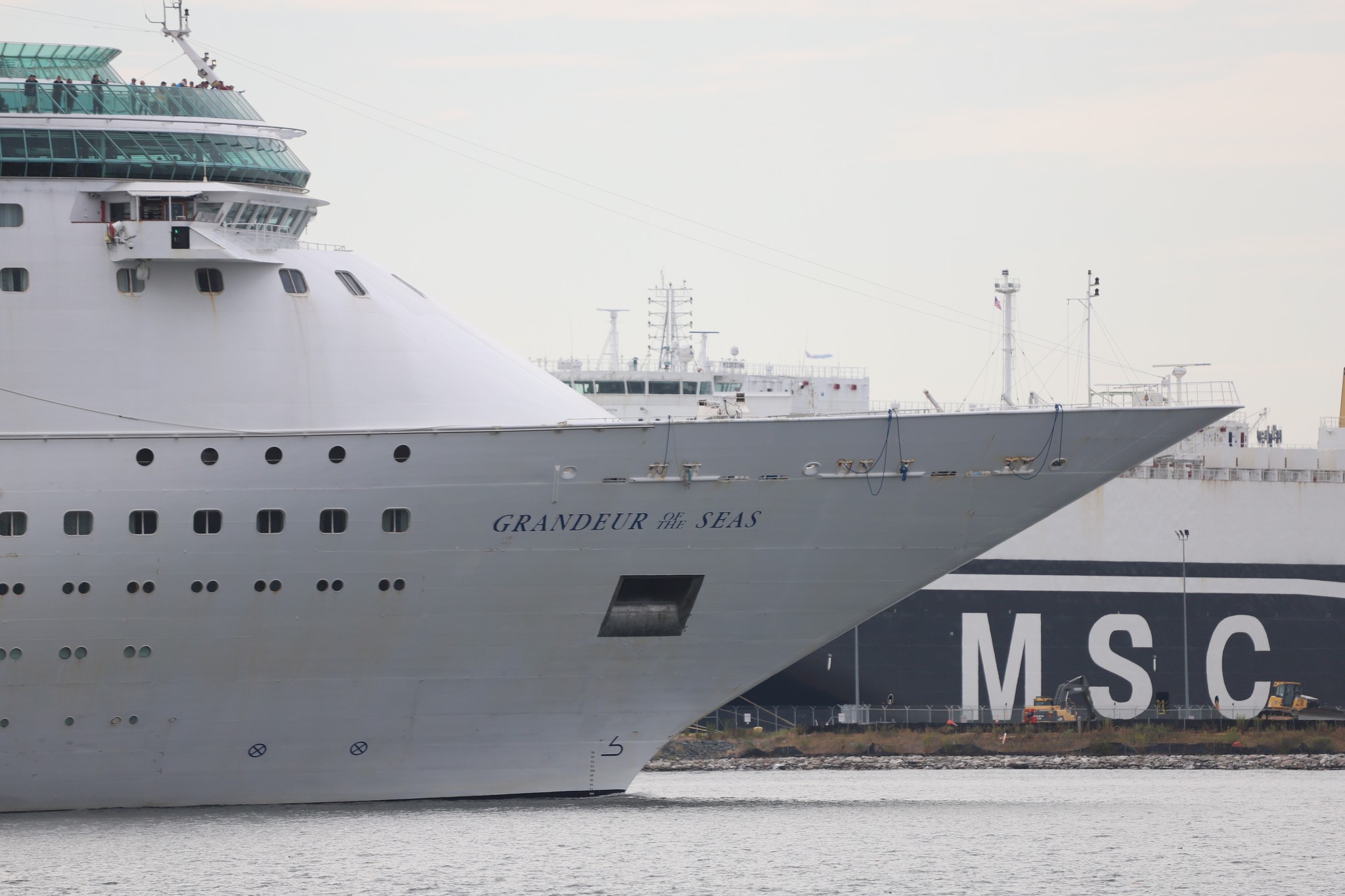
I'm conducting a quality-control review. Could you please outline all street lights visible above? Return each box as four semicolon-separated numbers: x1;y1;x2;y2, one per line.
1174;527;1192;721
1004;703;1007;724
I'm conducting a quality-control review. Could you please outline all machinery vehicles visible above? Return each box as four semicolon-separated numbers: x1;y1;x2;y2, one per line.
1023;674;1102;731
1268;679;1319;715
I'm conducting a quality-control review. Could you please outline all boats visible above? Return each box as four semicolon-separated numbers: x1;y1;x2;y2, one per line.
533;272;1344;730
0;1;1245;811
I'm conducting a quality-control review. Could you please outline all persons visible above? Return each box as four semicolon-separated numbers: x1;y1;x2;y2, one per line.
214;208;220;213
1;136;257;178
0;74;246;114
172;208;181;219
147;212;154;220
246;215;252;223
